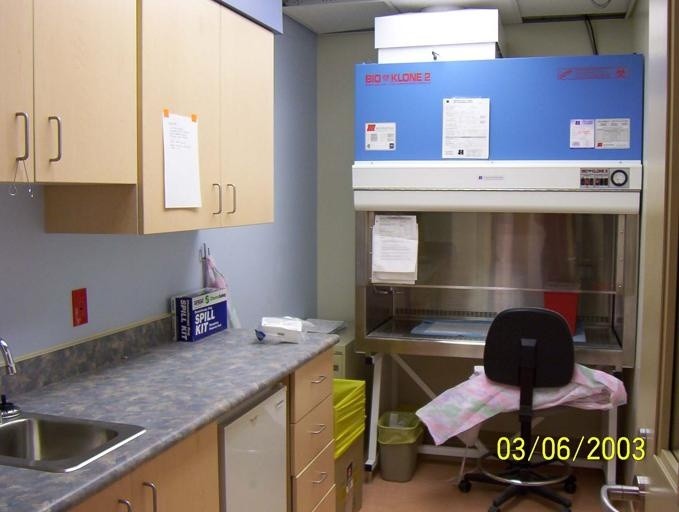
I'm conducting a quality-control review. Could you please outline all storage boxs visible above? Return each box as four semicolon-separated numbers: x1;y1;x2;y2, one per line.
374;8;506;64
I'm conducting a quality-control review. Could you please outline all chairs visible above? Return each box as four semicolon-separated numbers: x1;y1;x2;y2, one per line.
458;307;577;512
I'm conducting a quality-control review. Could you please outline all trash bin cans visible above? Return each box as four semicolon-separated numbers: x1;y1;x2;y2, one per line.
377;411;424;482
334;378;366;512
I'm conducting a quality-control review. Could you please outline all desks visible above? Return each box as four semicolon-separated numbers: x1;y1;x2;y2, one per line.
362;317;624;485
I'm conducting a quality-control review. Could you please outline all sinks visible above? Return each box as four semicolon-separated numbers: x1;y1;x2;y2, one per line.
1;410;145;473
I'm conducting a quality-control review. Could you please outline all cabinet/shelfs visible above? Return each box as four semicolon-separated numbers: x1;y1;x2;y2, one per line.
44;0;274;236
0;0;138;185
289;350;337;512
68;423;221;512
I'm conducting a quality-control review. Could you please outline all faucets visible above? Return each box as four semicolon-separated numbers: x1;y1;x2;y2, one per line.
0;338;18;377
